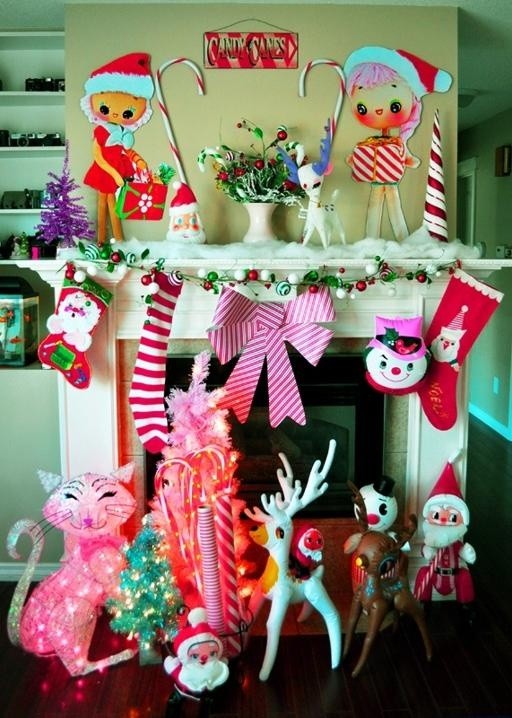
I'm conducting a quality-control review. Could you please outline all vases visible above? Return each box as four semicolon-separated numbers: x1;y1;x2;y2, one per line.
241;199;278;243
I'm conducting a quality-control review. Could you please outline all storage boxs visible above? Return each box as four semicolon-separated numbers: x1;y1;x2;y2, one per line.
1;275;42;367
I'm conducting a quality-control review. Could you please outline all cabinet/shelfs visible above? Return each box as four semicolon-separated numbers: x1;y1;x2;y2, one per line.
0;26;67;368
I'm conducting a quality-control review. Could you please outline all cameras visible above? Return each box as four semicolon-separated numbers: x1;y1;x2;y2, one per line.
25;78;55;91
37;134;61;146
56;79;65;91
10;133;35;147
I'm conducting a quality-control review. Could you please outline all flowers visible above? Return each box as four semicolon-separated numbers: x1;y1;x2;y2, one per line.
198;118;310;205
